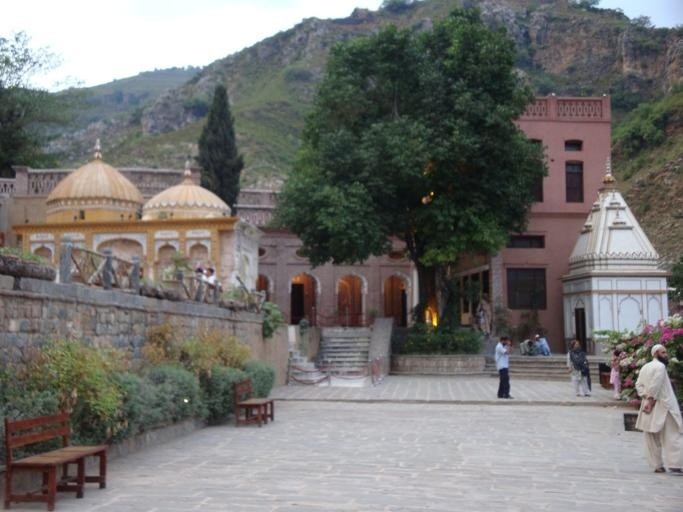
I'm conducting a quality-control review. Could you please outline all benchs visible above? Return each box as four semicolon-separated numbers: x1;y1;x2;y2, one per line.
232;376;273;427
3;408;109;511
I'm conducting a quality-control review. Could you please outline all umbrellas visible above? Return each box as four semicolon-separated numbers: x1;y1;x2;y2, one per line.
585;360;591;391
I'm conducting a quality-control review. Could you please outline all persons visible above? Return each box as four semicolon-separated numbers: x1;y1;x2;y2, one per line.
609;350;623;400
634;344;683;473
534;333;550;356
194;267;207;286
566;339;591;398
492;335;514;399
205;269;221;295
297;312;309;345
519;338;536;356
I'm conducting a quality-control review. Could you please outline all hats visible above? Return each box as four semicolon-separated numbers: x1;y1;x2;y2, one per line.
650;344;662;356
535;334;539;338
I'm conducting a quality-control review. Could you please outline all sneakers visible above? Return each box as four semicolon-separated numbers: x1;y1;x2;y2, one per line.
655;467;683;475
